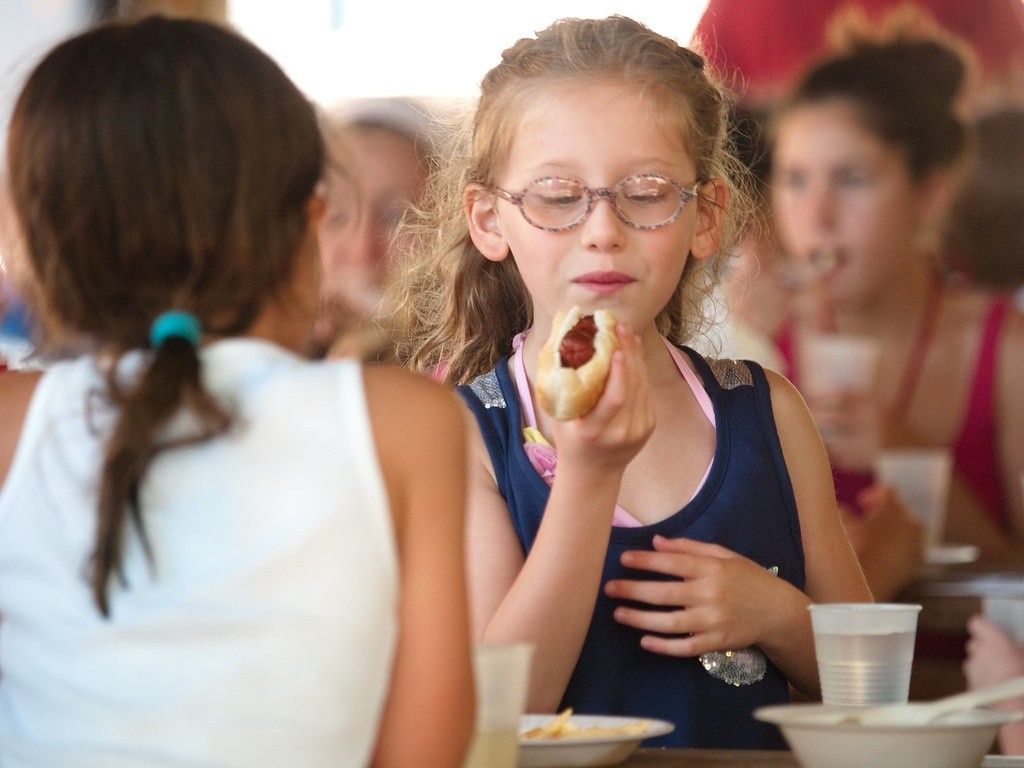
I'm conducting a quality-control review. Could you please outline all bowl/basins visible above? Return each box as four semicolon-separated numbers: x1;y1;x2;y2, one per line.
753;704;1024;768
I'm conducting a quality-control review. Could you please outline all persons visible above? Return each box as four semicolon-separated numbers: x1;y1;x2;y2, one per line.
399;15;875;751
0;0;474;767
681;2;1024;756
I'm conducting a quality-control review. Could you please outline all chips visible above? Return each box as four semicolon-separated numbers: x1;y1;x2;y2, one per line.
522;706;648;741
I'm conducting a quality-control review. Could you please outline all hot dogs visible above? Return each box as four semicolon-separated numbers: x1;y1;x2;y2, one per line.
533;306;619;420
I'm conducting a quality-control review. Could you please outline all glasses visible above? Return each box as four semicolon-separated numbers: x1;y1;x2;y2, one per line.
480;171;704;233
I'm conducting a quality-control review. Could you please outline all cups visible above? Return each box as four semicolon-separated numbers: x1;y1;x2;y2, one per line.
981;584;1024;651
868;449;954;547
809;601;920;707
466;640;539;768
798;337;879;467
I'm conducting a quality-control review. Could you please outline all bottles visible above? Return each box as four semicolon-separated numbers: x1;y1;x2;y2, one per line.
914;543;981;702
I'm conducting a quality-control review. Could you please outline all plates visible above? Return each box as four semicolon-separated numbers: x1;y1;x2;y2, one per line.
516;714;677;768
